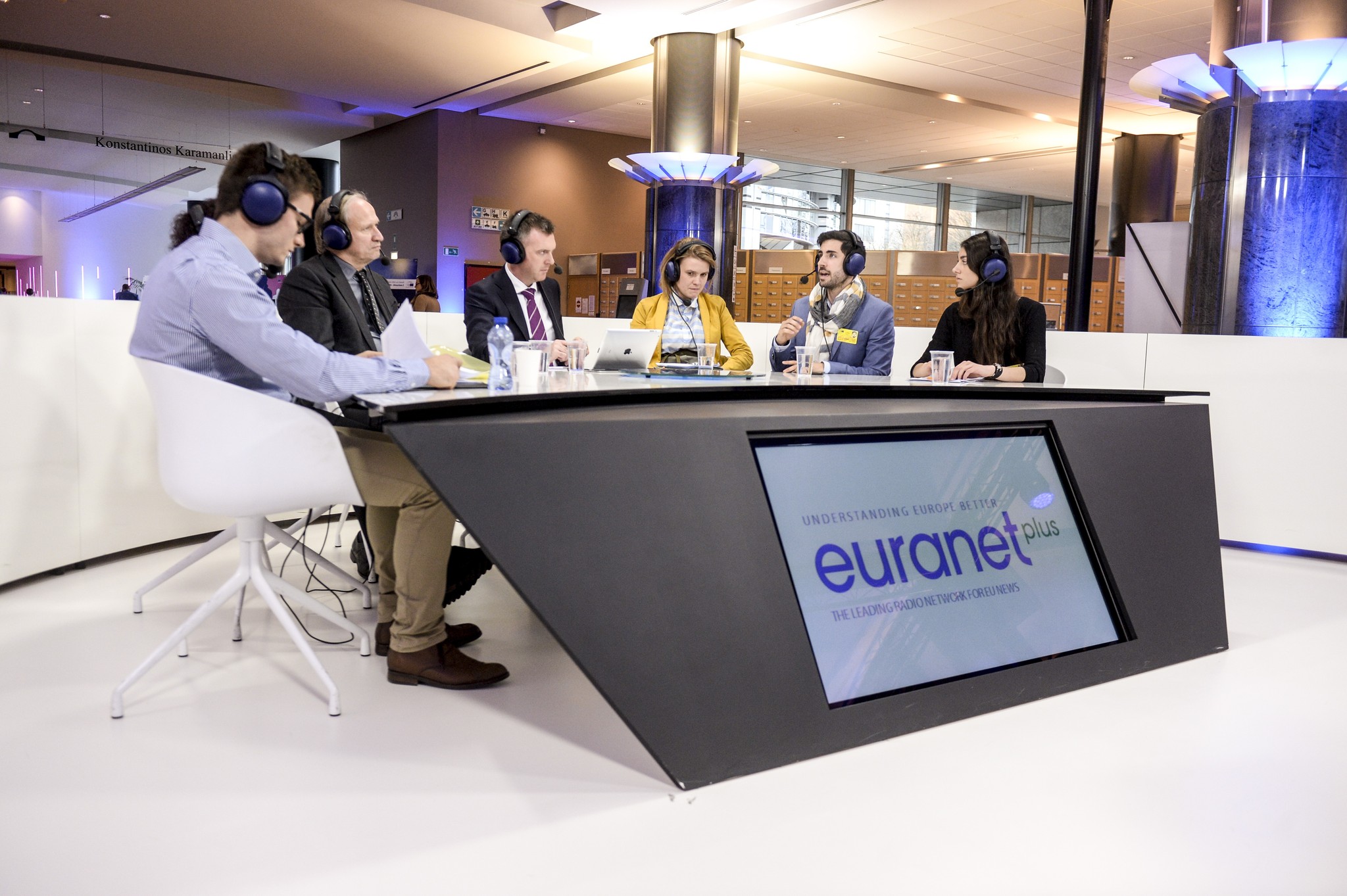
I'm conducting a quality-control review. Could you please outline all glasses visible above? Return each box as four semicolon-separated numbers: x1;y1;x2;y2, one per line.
287;201;314;235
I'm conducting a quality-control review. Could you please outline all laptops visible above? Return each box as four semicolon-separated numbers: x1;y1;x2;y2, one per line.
583;329;662;372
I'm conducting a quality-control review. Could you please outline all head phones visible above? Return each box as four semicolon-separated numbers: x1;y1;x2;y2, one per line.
979;230;1009;285
665;240;716;283
189;204;205;235
238;141;292;226
321;189;353;251
500;209;534;264
814;230;866;276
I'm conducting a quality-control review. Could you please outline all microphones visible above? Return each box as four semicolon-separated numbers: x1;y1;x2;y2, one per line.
554;262;563;275
664;270;692;307
800;270;816;284
955;269;1001;297
260;267;276;279
262;263;284;274
379;249;391;266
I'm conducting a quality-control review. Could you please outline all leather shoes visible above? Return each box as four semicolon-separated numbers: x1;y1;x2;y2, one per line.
387;639;510;690
375;619;482;656
349;536;370;579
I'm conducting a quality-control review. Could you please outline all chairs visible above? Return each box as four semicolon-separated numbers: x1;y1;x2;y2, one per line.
108;351;371;722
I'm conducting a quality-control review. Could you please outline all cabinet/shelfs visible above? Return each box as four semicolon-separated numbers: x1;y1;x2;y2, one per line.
565;252;1126;334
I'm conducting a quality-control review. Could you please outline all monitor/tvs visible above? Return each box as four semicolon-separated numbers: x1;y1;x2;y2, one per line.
748;423;1132;710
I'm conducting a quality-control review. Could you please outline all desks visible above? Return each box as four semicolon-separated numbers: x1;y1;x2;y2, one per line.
344;367;1228;795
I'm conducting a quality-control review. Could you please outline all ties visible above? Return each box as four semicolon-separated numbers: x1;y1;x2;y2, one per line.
521;288;547;340
355;270;387;335
256;275;272;300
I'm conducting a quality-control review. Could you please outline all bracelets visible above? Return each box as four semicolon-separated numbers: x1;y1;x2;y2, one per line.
989;363;1003;379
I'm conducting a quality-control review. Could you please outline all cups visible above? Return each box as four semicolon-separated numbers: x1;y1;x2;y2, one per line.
929;351;955;383
512;341;529;376
697;343;718;369
515;349;542;389
566;340;586;371
794;346;816;374
528;340;553;373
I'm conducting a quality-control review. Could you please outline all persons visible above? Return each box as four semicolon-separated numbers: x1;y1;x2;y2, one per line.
26;288;34;296
128;141;510;689
410;275;441;313
169;197;284;305
277;190;408;584
769;230;895;377
115;283;140;301
631;237;754;372
1;288;8;295
910;230;1046;383
462;210;589;368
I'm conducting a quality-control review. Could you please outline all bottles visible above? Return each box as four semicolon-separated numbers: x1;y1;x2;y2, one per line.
487;317;514;392
